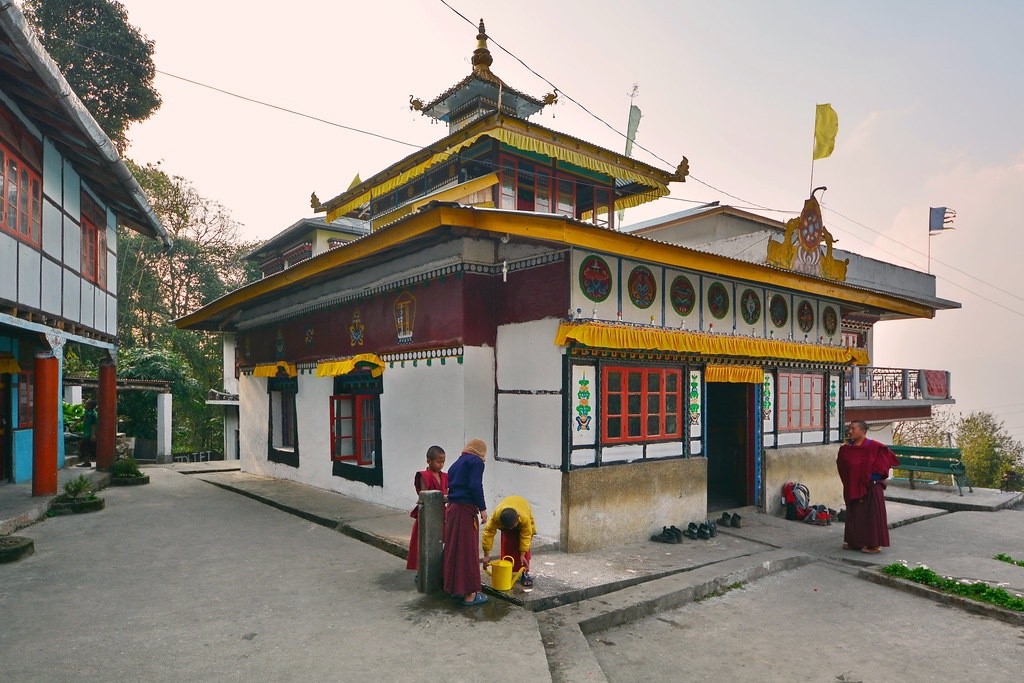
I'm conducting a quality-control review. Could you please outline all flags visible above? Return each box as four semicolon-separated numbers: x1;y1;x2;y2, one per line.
930;206;957;230
814;102;839;161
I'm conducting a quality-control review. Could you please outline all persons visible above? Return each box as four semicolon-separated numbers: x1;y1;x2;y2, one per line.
482;497;536;589
836;420;896;553
444;439;487;605
410;446;449;581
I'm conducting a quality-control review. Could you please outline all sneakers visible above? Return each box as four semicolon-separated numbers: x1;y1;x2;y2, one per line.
667;525;682;544
697;523;710;539
650;526;678;544
683;522;698;539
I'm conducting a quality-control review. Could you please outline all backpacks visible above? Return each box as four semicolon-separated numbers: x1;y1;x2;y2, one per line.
781;481;810;520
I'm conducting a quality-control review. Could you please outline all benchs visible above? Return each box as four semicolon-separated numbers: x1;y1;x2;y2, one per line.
885;445;973;496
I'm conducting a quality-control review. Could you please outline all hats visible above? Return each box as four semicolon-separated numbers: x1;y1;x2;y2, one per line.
460;439;487;463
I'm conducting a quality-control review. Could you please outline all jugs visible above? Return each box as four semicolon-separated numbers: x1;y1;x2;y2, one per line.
484;555;526;590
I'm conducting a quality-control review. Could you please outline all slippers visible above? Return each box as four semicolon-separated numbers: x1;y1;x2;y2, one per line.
461;592;489;606
450;593;464;600
520;575;533;587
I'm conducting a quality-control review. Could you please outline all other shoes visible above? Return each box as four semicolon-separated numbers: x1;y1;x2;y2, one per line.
842;542;863;550
862;545;881;554
705;520;719;537
731;512;742;528
75;462;92;467
717;511;732;527
803;504;846;526
